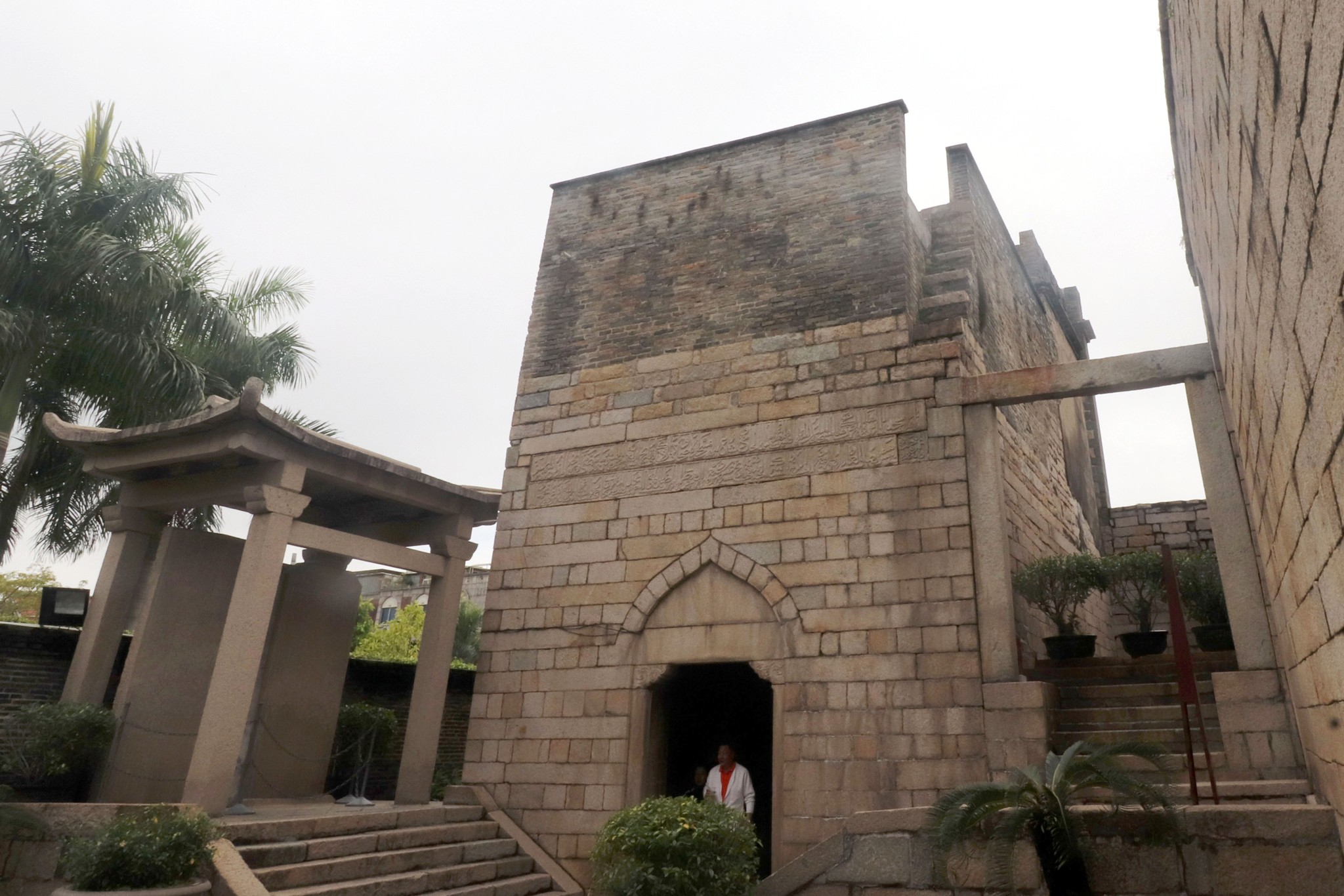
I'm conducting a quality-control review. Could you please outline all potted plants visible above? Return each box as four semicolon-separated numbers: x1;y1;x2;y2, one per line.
1155;548;1234;649
52;810;221;895
1018;549;1108;662
1088;548;1169;659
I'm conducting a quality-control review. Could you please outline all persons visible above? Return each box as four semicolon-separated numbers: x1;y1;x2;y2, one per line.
702;745;756;820
688;766;707;801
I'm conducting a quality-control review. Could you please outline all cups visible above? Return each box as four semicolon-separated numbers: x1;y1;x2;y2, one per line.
708;787;716;796
